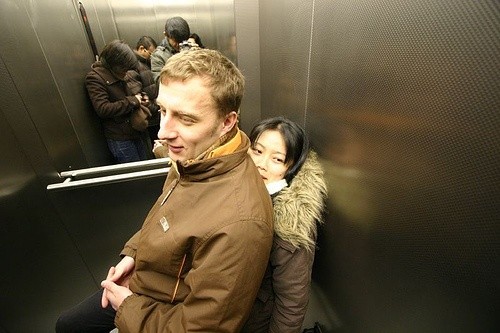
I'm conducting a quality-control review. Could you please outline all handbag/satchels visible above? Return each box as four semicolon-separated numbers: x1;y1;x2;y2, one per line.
130;104;152;132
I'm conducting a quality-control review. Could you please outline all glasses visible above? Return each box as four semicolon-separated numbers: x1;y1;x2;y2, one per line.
144;49;151;58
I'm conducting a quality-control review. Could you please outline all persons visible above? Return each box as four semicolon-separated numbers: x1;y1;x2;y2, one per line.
150;16;191;89
238;116;329;333
55;49;274;333
85;39;152;164
131;36;161;158
